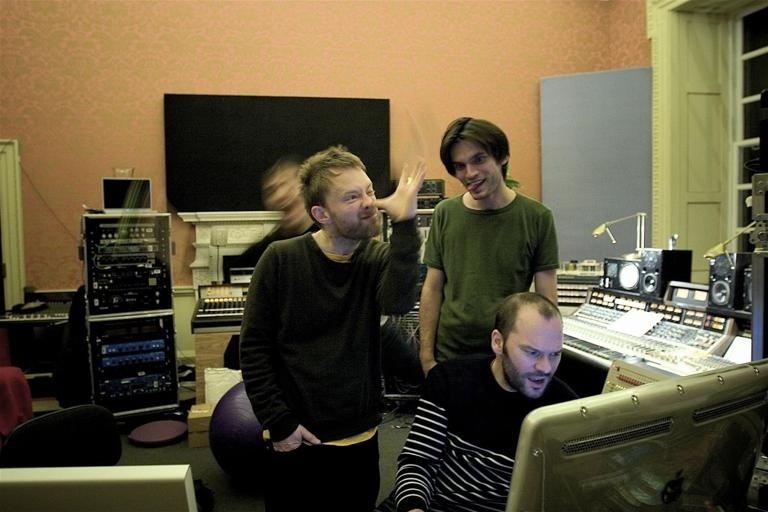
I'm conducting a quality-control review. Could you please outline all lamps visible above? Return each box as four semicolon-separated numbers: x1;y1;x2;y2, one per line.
591;212;647;260
702;221;756;259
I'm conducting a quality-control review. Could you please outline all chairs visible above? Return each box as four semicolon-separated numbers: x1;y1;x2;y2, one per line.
2;405;217;510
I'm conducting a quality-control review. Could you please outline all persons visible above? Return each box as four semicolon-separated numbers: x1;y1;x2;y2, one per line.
417;117;561;383
242;151;404;367
237;146;428;511
375;291;581;512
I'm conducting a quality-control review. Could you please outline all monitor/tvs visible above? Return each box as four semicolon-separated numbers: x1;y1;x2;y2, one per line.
164;94;390;213
506;358;768;512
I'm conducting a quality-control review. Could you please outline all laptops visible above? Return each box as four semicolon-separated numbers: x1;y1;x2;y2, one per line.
101;177;158;214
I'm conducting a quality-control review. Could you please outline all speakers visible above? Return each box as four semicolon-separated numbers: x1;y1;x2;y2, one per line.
708;252;753;310
603;248;692;298
